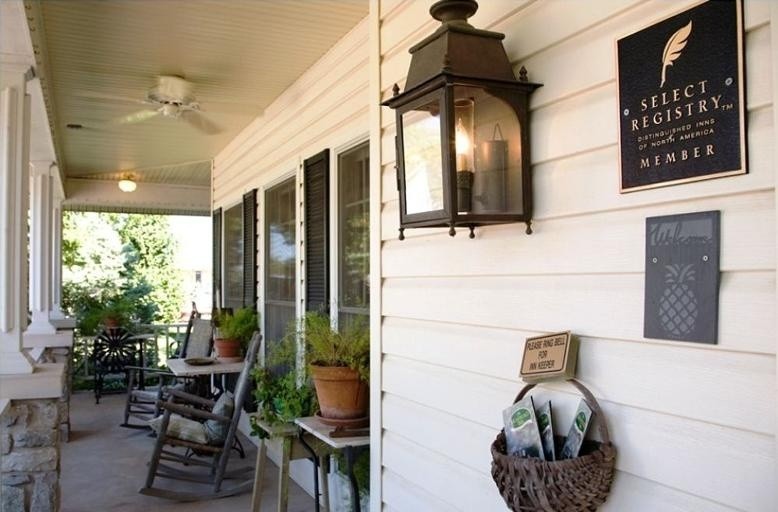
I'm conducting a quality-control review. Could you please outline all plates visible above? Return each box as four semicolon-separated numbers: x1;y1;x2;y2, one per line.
185;358;216;366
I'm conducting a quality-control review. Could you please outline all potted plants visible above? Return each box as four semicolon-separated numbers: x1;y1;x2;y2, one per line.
213;302;259;358
84;300;140;330
267;295;370;427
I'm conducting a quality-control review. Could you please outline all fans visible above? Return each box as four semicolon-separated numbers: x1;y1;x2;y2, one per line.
72;73;267;136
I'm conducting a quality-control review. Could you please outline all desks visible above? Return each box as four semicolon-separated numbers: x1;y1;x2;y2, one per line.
82;334;154;395
251;414;369;512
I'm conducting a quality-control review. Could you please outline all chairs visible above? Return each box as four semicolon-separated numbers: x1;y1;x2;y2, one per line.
93;327;137;403
118;308;263;502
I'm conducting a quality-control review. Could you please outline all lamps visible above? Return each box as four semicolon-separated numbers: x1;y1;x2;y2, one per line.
117;173;137;192
379;0;544;240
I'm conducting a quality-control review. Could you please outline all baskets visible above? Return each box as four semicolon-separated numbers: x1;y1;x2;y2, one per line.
491;375;616;510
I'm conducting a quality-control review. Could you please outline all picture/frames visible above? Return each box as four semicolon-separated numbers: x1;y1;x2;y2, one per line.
616;0;748;193
